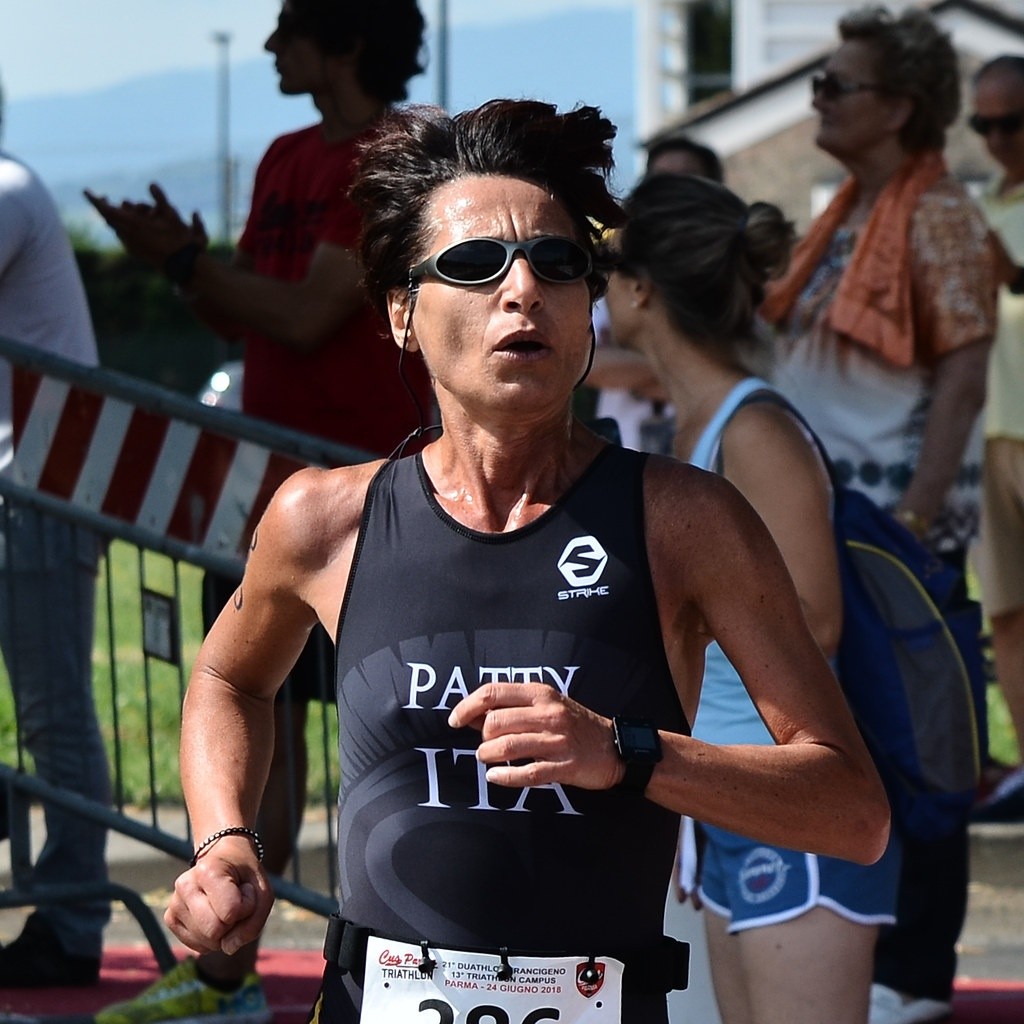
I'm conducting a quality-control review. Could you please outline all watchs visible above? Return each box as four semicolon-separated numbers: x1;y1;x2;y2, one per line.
609;714;663;795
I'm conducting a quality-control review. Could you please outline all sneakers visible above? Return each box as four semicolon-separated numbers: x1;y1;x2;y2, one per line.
91;955;273;1024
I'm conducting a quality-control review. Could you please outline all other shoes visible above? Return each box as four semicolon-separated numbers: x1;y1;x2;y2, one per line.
0;913;100;990
970;766;1024;824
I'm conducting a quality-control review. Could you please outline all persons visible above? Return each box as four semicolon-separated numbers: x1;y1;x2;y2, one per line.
0;149;116;989
950;50;1024;824
581;134;728;459
164;97;890;1024
605;170;907;1024
759;4;997;1023
87;0;426;1024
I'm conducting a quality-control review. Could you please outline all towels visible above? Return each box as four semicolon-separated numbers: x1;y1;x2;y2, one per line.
754;147;949;371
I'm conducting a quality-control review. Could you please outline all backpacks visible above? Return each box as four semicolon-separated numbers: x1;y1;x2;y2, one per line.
715;388;990;849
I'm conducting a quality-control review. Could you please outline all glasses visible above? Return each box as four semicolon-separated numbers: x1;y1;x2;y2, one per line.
969;104;1024;138
810;72;878;102
409;231;608;289
276;13;312;44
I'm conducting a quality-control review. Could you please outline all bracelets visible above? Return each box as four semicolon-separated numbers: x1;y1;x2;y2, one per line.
188;827;264;866
1010;266;1024;294
900;509;926;536
